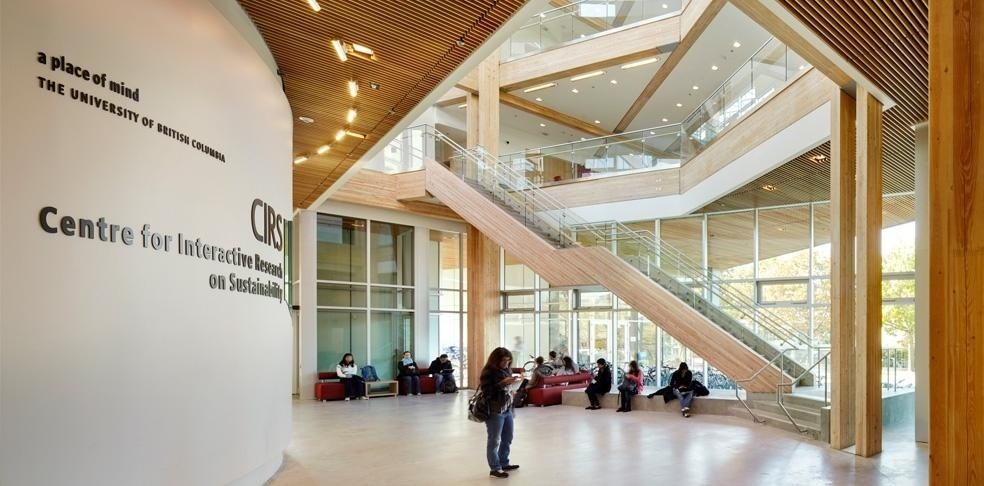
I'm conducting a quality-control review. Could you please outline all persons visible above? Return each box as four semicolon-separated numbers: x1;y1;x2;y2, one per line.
669;362;694;417
425;354;459;396
335;353;368;401
553;356;578;386
615;360;644;412
479;346;519;479
584;358;611;410
548;350;562;374
516;356;552;393
397;351;421;397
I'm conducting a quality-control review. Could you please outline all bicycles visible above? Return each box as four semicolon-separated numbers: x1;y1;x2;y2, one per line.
577;358;736;391
522;352;567;371
444;345;468;370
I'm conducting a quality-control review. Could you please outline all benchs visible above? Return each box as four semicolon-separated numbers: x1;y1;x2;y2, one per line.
512;368;525;374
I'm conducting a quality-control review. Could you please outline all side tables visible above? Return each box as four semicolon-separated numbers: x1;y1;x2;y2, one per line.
364;380;399;399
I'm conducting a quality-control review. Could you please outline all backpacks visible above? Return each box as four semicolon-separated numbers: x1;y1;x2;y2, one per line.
440;375;456;393
362;366;377;381
692;380;709;396
512;379;528;408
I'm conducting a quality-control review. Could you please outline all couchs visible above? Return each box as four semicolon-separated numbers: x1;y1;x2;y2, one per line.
397;369;436;396
314;373;344;402
525;370;592;408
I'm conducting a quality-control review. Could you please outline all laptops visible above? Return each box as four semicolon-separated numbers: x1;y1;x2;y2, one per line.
442;369;454;374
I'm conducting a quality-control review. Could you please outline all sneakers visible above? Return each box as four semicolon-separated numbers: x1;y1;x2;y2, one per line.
585;406;601;410
490;470;508;477
616;408;630;412
503;465;519;469
681;407;690;417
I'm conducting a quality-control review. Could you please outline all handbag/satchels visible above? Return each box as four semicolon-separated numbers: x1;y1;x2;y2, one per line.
468;385;490;423
618;381;636;391
